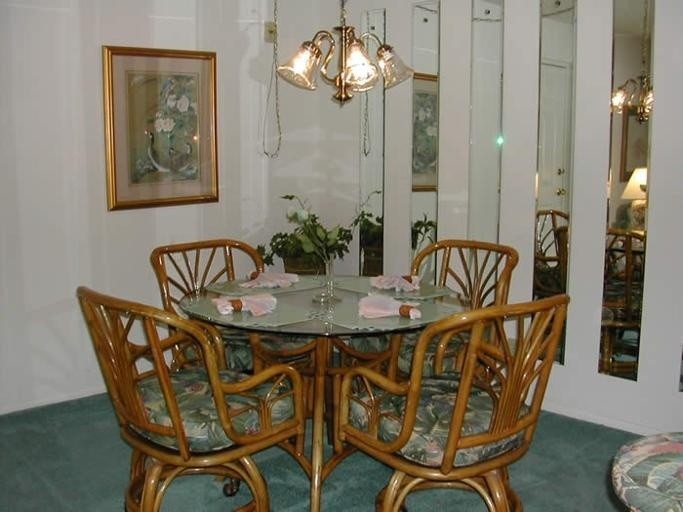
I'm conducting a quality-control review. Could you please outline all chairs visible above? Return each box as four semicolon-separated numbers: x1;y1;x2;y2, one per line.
535;210;569;295
323;294;568;511
75;285;311;510
601;230;646;376
153;239;308;375
337;236;516;386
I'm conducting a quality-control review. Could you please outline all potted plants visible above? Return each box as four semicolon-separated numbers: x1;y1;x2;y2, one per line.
249;194;357;274
355;189;385;277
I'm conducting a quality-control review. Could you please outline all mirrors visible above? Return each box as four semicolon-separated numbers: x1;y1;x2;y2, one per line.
355;7;388;281
596;0;655;384
532;0;571;366
410;0;440;289
468;0;499;295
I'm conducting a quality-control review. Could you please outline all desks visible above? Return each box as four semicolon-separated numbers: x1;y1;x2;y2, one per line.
181;275;472;497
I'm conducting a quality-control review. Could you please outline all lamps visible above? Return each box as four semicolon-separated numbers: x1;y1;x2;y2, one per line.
618;165;648;238
279;0;415;110
608;47;655;122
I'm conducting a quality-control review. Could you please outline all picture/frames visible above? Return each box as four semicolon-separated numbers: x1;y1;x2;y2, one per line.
620;108;650;182
411;71;441;193
100;44;219;213
